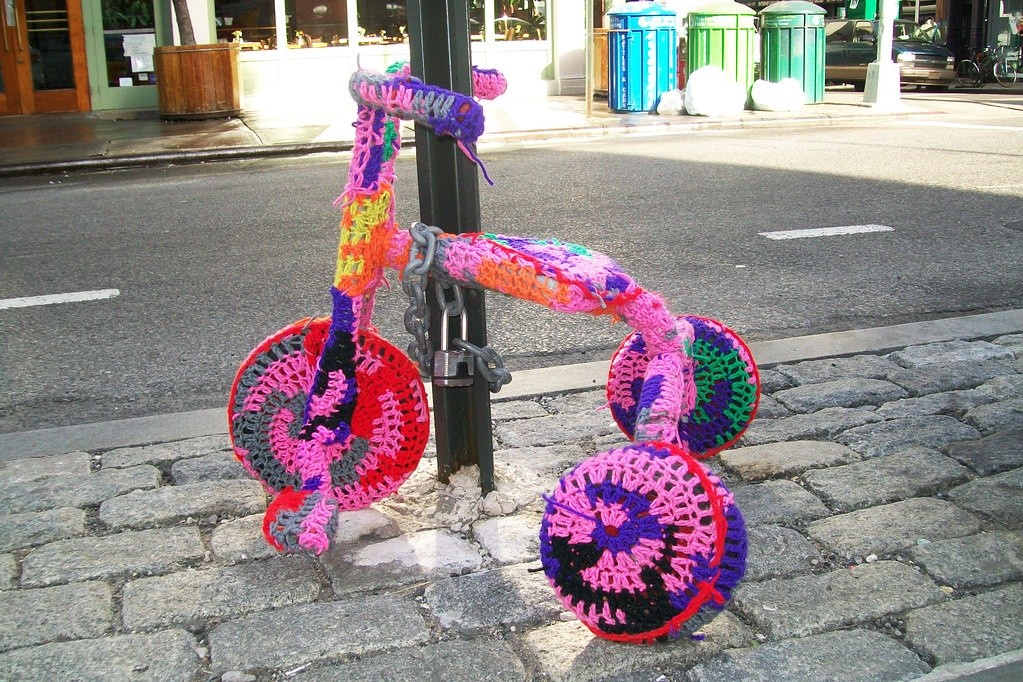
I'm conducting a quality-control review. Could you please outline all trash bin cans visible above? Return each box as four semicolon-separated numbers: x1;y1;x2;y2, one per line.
686;1;758;110
837;7;845;19
606;0;679;113
757;0;828;106
846;0;876;20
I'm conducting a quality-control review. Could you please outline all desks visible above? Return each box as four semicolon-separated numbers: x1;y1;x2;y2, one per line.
472;34;507;40
240;42;261;50
331;37;382;45
289;42;327;48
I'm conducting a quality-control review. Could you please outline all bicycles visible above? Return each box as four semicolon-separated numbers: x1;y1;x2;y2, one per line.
957;41;1017;89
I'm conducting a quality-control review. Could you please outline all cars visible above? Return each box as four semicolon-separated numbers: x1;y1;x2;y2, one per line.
824;18;957;91
